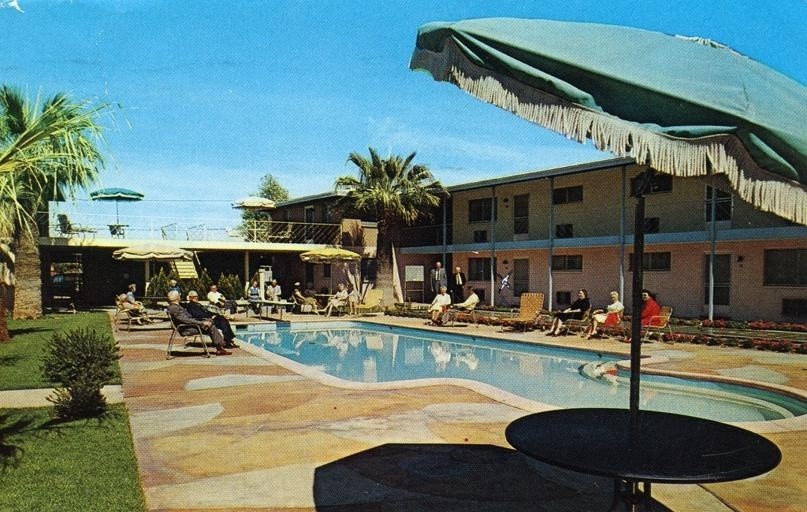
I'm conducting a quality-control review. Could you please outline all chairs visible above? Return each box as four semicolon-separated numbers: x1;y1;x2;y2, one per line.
115;293;145;332
226;222;297;243
54;214;98;239
628;306;674;344
165;311;211;359
354;289;384;316
500;292;544;332
556;303;592;337
428;296;476;327
156;286;357;322
588;306;625;340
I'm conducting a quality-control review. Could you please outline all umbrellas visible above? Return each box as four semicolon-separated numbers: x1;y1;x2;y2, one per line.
90;187;144;225
113;243;194;297
411;16;807;411
231;197;276;240
299;246;362;294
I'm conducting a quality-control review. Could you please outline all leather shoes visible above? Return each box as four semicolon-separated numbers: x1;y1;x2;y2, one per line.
226;341;239;348
217;350;232;355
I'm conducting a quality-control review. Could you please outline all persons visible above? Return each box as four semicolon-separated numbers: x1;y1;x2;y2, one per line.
545;288;590;337
118;279;362;355
623;290;660;343
583;290;623;340
428;261;480;327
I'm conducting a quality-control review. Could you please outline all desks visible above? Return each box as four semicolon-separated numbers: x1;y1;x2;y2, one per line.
106;224;129;239
504;407;783;512
